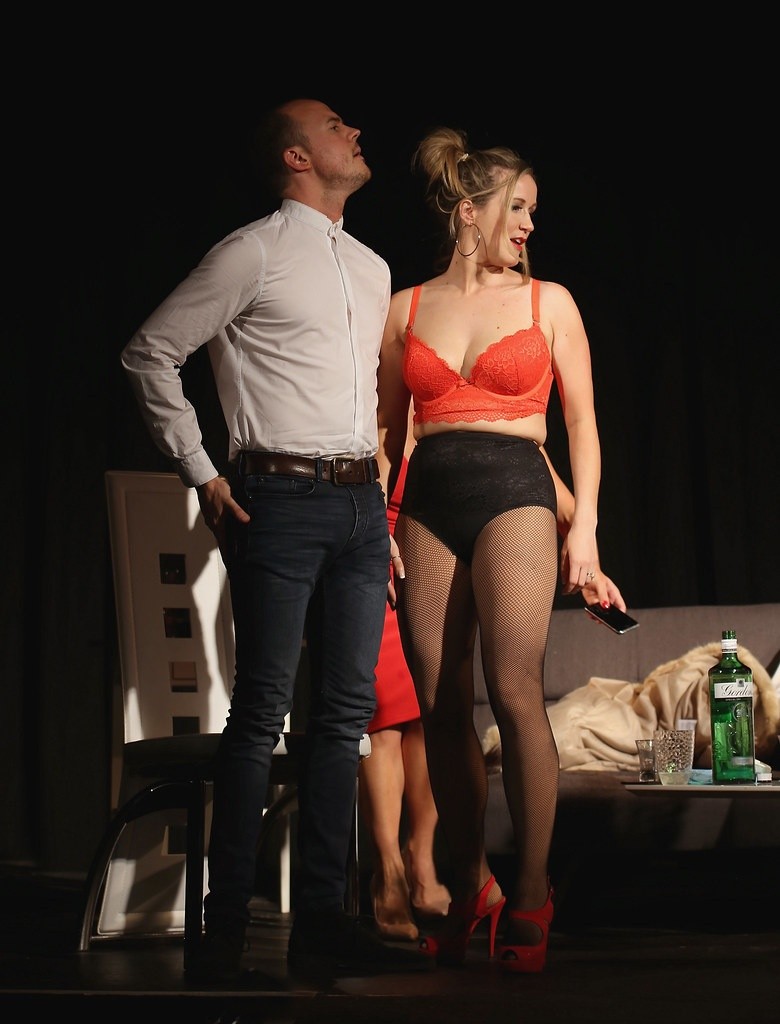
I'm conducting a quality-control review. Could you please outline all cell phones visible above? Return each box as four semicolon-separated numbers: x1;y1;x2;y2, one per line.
585;601;639;635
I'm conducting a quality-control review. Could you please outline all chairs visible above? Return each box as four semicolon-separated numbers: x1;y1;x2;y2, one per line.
69;469;362;974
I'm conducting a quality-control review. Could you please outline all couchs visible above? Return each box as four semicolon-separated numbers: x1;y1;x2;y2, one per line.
472;602;780;860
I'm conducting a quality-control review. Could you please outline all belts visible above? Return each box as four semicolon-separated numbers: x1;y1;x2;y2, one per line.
242;451;380;485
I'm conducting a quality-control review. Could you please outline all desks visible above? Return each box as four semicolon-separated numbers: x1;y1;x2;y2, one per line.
618;779;780;798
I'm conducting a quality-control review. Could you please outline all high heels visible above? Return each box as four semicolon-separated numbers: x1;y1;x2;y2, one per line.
400;850;451;916
369;872;417;941
498;876;555;973
418;875;506;958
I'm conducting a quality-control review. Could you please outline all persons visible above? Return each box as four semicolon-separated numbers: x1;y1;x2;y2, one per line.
378;129;626;973
358;427;450;939
123;99;392;966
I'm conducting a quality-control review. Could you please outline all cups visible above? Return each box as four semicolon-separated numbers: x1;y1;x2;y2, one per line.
634;739;658;782
652;730;695;785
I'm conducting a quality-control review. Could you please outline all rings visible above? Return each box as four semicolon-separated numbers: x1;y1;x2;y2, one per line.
392;555;402;560
587;572;595;578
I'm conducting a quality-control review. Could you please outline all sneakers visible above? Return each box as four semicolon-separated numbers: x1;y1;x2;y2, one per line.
287;906;402;974
183;927;256;986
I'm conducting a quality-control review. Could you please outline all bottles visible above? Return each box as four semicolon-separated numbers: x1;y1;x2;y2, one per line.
708;630;756;785
